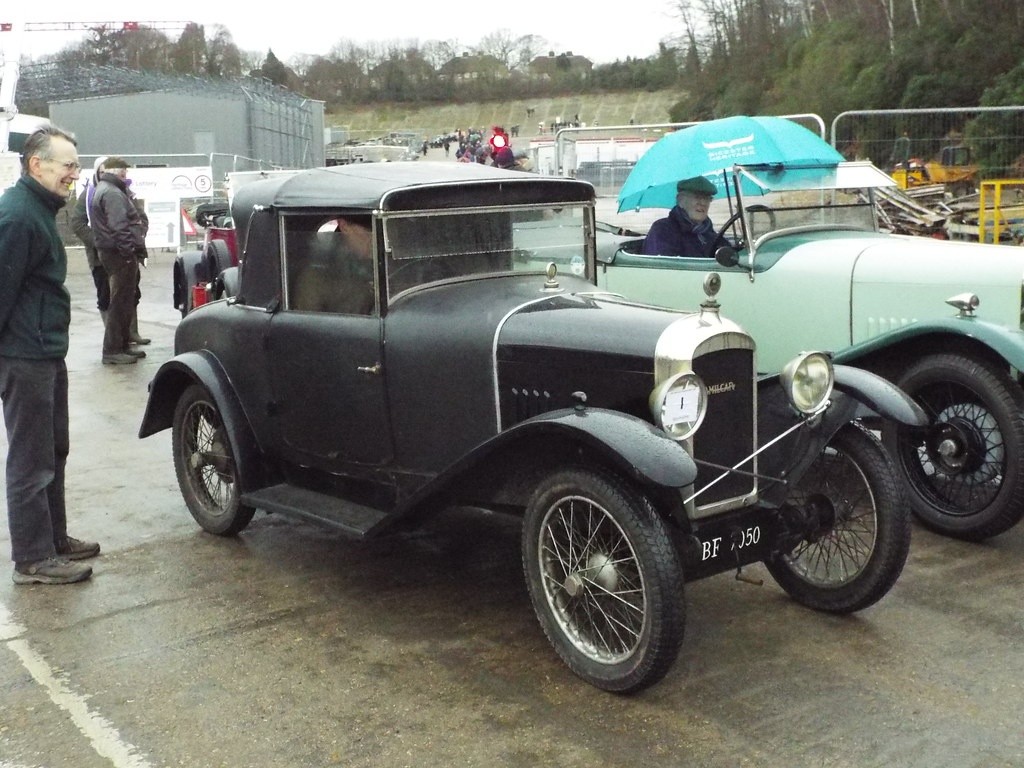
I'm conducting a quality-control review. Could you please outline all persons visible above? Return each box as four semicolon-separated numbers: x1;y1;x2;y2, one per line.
91;157;146;366
526;107;635;136
419;124;525;173
71;156;150;344
640;175;734;258
290;213;413;314
0;126;101;584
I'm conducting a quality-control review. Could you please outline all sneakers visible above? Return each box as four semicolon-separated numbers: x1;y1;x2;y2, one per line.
12;556;92;586
102;352;137;364
55;535;101;560
125;348;146;358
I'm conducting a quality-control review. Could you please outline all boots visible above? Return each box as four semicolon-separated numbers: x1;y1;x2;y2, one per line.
129;306;151;345
100;311;107;325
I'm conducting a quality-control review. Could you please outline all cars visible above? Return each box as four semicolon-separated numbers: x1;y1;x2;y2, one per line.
172;202;238;320
514;157;1024;541
138;159;931;694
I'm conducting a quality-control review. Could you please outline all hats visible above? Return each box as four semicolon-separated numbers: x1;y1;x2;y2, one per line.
340;214;372;228
677;176;718;195
496;146;513;165
105;157;132;169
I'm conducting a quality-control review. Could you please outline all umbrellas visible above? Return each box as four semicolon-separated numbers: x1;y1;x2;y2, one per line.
615;113;847;245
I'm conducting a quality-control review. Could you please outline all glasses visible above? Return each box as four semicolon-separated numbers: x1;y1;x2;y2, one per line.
44;158;81;174
